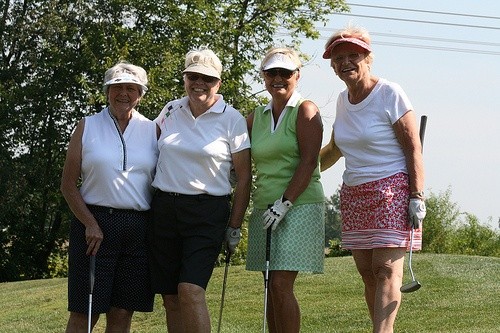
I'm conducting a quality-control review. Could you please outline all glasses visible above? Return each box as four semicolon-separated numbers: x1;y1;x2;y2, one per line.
266;68;295;78
187;72;214;82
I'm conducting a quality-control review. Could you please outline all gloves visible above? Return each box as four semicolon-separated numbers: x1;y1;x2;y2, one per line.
222;225;241;260
407;198;426;229
260;194;294;230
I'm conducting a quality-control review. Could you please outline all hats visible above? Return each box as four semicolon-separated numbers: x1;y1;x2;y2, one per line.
261;53;298;71
321;34;371;59
182;61;221;79
102;74;149;92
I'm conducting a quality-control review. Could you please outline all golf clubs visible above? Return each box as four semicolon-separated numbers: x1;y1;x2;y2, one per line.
87;252;96;333
218;243;232;333
262;208;272;333
399;113;427;292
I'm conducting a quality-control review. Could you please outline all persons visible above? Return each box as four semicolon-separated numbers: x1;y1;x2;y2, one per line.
246;47;325;333
60;63;161;333
151;49;251;333
319;20;426;333
